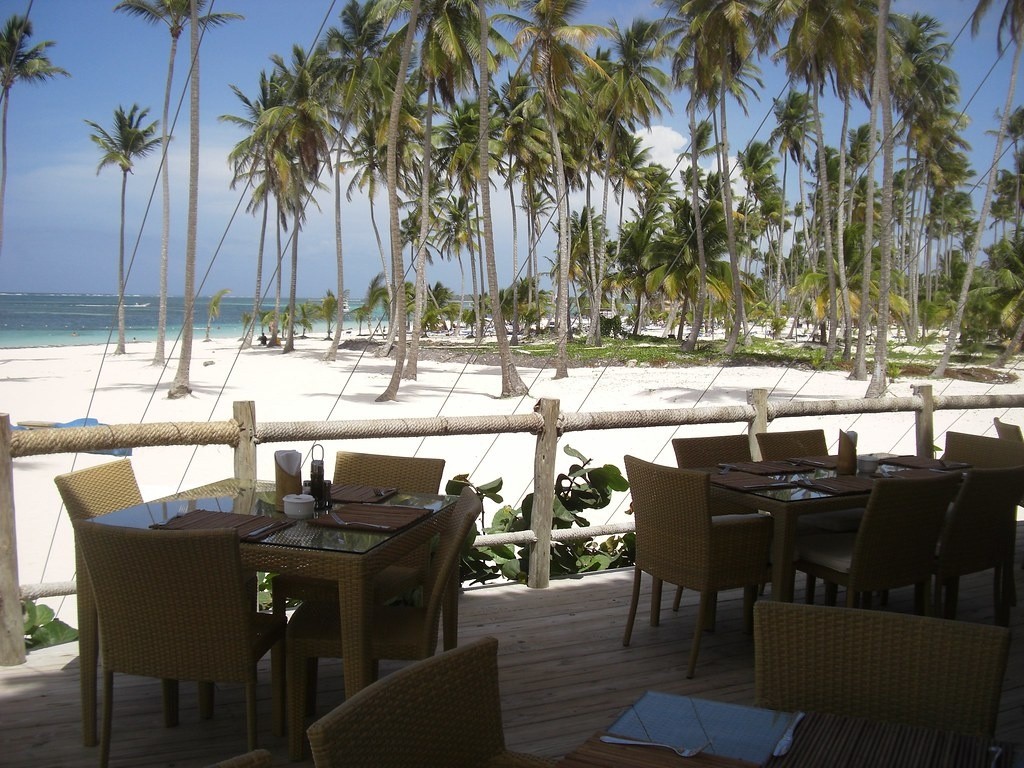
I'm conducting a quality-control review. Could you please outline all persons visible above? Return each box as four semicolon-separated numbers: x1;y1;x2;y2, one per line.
260;333;267;346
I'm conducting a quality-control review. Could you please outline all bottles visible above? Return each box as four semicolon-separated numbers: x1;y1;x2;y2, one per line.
303;481;311;494
324;480;332;509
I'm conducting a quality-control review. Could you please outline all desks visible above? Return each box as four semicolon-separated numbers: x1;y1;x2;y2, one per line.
686;452;972;605
556;689;1024;768
74;477;459;747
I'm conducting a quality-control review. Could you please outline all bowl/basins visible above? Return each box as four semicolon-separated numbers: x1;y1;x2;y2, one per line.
858;454;880;473
283;494;315;519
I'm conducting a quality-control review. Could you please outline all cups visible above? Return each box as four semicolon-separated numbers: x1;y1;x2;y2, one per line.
312;444;324;510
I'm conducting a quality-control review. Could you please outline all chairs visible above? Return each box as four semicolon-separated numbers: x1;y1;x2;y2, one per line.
622;455;774;679
946;431;1024;607
285;486;483;763
933;464;1024;628
305;636;559;768
752;599;1011;738
756;430;867;604
52;459;215;724
672;435;819;635
271;451;447;616
994;417;1024;442
70;515;288;768
766;471;964;616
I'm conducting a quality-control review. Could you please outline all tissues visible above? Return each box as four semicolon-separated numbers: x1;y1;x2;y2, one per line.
272;450;302;512
835;430;858;475
